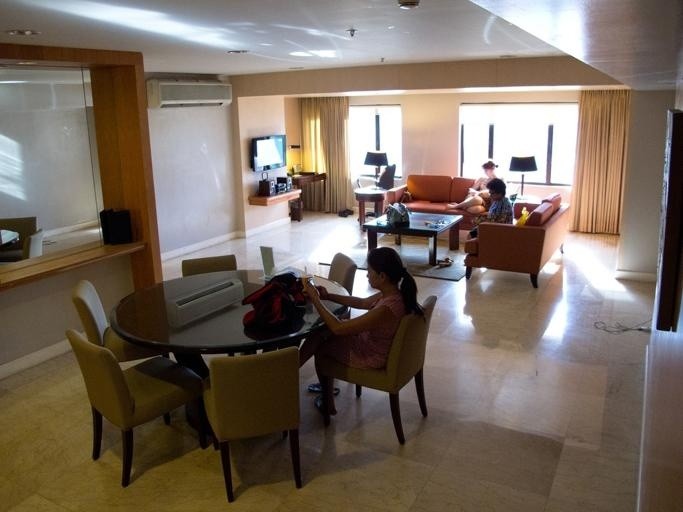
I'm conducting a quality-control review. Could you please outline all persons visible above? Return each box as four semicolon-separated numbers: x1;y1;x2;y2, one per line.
446;160;499;215
304;246;428;415
466;178;513;239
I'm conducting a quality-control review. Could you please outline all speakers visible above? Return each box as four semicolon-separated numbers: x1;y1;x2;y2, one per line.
260;180;276;196
278;177;293;192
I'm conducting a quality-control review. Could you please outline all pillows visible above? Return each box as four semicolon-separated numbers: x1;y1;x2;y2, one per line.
542;194;561;213
450;177;474;201
407;175;452;202
524;202;553;227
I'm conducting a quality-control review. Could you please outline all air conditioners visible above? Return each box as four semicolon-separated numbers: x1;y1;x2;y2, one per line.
146;78;232;109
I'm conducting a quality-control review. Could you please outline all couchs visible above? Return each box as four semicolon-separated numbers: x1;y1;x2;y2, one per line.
387;185;475;230
465;201;570;288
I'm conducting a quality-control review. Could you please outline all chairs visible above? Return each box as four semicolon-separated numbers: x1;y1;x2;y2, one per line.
0;216;36;262
0;228;42;265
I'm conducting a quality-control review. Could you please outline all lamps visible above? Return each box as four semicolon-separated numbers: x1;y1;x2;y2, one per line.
509;157;537;195
364;152;388;189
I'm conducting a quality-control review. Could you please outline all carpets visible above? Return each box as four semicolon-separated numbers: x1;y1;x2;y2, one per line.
319;234;475;281
0;342;564;511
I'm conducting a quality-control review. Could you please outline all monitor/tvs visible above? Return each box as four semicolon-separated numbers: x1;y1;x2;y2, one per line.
252;134;289;172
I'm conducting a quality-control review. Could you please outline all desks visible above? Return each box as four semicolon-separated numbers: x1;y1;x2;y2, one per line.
353;186;385;230
0;229;21;249
292;173;326;222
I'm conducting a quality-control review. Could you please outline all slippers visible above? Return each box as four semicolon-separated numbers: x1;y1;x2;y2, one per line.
314;394;325;410
437;257;453;267
307;382;339;395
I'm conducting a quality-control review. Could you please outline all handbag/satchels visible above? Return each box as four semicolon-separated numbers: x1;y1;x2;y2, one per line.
241;274;307;335
386;202;409;228
401;190;413;203
